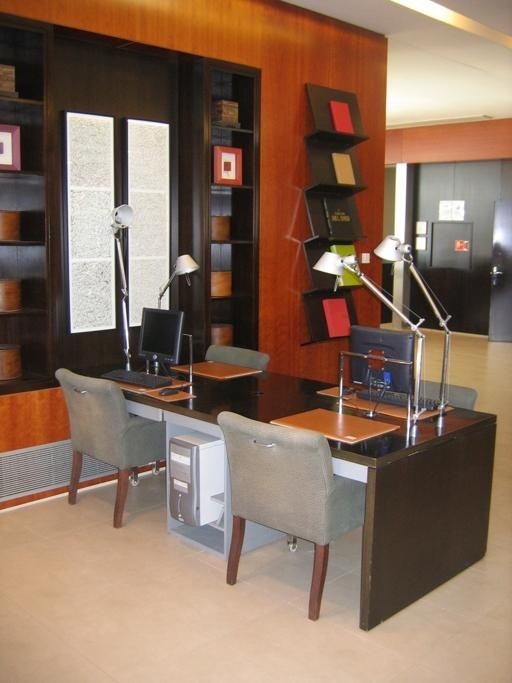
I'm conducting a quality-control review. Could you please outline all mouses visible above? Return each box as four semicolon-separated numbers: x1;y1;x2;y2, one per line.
159;388;178;396
341;386;356;395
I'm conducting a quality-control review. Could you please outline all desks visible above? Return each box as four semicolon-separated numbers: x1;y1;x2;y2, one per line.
100;359;499;631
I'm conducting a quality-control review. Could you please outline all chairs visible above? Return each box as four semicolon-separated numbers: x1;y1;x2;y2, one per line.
53;365;168;528
202;342;270;372
213;408;369;620
413;380;479;410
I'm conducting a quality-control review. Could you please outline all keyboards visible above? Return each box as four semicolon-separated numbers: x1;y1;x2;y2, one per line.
357;391;441;411
101;367;172;389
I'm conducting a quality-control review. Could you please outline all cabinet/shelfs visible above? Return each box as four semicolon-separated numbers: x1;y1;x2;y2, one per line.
185;61;261;351
0;9;59;394
300;79;370;347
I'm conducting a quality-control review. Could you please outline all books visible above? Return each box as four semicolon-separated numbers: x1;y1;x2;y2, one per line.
330;245;363;287
324;199;355;237
331;153;357;185
322;298;351;339
330;101;354;135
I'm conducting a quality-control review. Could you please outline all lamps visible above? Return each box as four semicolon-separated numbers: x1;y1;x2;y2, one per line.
314;248;432;446
373;236;454;436
108;200;134;371
158;252;201;308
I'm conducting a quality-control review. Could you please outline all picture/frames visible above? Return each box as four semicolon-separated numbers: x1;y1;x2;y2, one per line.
212;143;244;185
0;121;23;172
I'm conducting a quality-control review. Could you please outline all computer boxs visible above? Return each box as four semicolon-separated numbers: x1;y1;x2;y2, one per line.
167;433;224;528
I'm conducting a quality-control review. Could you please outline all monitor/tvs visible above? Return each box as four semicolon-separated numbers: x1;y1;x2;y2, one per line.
136;307;185;365
351;324;415;394
